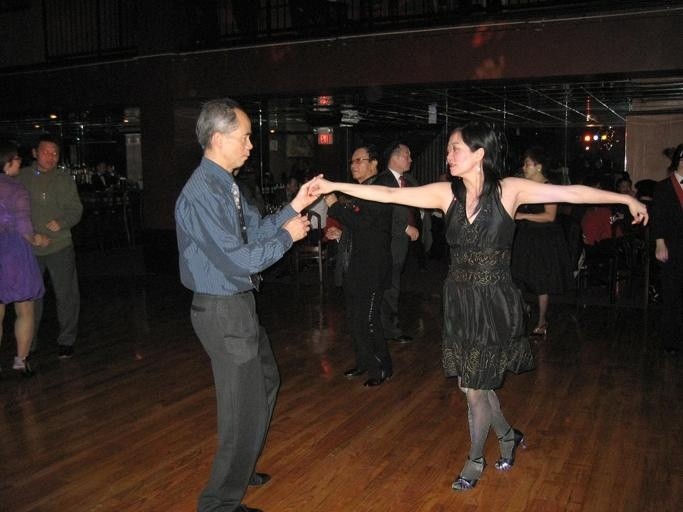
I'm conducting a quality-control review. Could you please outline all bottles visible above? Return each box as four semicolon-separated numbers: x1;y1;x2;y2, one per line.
260;181;284;216
59;163;94;185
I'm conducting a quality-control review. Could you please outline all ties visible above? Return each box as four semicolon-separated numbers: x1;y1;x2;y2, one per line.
231;182;261;293
399;176;414;225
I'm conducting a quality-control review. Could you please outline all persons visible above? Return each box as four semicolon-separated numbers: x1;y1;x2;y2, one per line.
308;122;653;491
167;100;323;512
0;148;52;377
236;172;309;217
91;162;116;190
323;142;454;388
511;144;682;359
14;139;84;361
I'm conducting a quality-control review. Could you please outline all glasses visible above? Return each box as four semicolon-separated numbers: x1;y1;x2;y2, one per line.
11;156;20;160
351;157;369;163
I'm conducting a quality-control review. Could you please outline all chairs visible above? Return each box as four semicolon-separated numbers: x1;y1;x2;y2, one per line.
297;207;339;293
609;215;655;301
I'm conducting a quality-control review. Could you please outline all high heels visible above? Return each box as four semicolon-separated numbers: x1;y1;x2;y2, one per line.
452;455;486;490
533;324;548;335
495;429;526;470
13;357;30;378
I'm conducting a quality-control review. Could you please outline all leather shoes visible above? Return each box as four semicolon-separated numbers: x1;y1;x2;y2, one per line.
363;381;379;387
58;345;72;359
234;505;262;511
344;368;361;377
248;472;271;488
